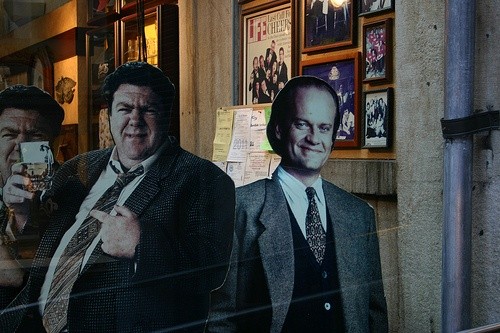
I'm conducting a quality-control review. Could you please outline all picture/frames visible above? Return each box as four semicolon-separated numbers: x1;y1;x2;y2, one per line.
238;0;397;160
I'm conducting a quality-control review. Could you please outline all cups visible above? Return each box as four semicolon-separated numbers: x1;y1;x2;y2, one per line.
20;142;53;192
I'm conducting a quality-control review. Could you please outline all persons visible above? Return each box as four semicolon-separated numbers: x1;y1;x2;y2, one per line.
366;98;387;136
3;60;236;333
0;86;66;312
366;27;385;76
248;41;288;103
327;66;354;139
205;76;389;333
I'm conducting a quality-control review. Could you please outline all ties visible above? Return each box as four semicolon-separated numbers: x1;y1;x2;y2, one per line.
0;203;9;237
305;187;327;265
42;161;144;333
